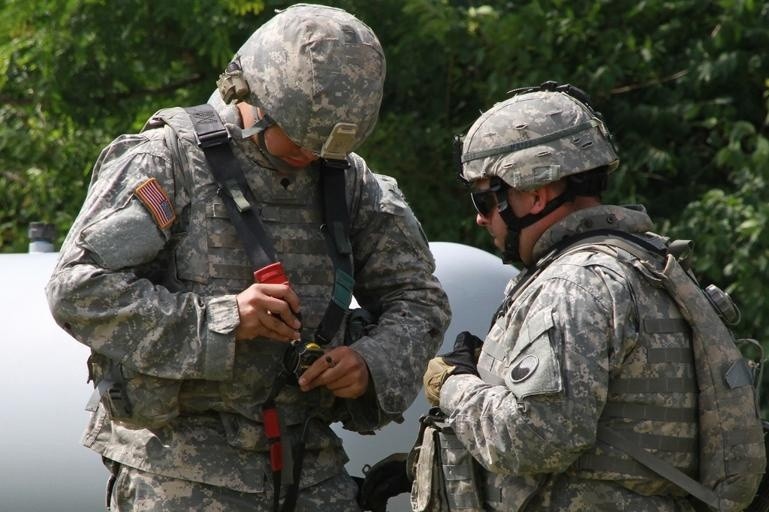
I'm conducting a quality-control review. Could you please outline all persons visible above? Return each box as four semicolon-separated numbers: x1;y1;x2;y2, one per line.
43;1;455;512
409;80;722;511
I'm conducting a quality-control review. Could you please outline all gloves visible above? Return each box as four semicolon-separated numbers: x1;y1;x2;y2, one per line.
423;329;481;407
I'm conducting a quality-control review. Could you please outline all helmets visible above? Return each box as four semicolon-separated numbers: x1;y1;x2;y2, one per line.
237;2;388;162
460;90;621;194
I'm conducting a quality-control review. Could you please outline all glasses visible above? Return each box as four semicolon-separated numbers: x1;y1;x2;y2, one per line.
469;186;498;221
